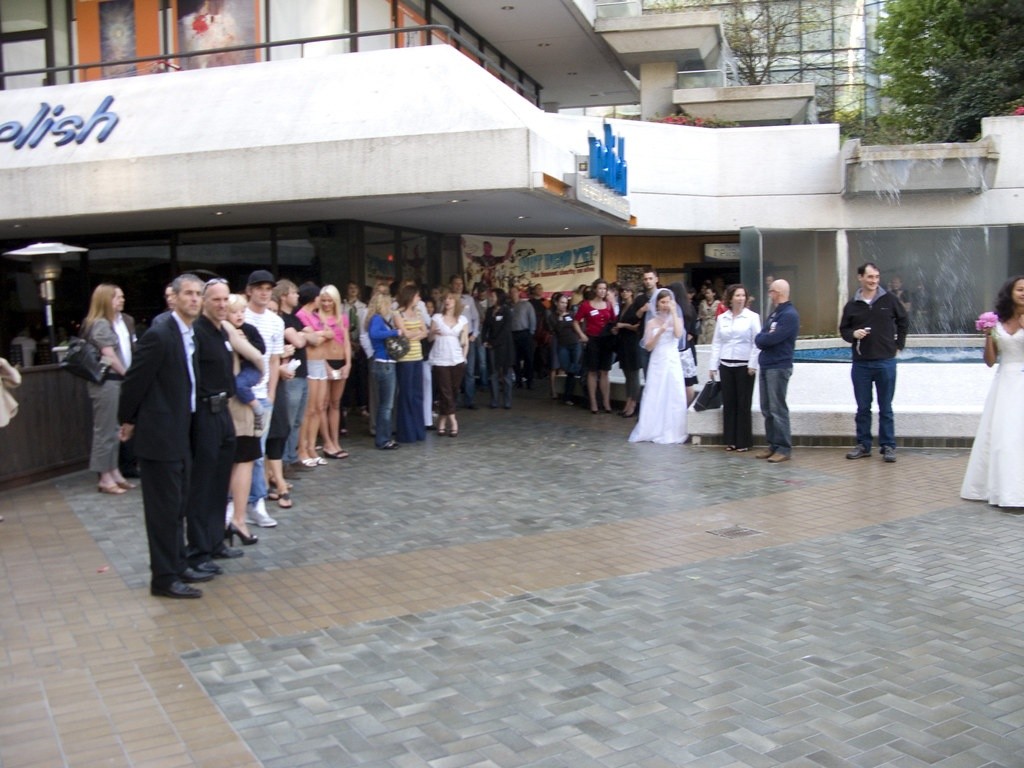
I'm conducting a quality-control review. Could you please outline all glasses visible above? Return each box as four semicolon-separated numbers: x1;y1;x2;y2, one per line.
203;277;230;294
619;288;630;293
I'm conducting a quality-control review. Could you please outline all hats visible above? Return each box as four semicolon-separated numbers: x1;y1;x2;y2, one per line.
248;271;279;287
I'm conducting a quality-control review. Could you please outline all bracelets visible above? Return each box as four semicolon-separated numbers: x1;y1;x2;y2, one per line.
985;334;992;338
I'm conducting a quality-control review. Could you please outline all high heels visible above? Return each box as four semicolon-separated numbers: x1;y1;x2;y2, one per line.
228;522;259;546
436;421;459;437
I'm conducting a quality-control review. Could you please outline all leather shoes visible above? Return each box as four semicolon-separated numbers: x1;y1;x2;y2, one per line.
591;401;612;414
196;560;223;574
617;406;635;419
152;580;202;597
213;546;244;557
180;568;215;582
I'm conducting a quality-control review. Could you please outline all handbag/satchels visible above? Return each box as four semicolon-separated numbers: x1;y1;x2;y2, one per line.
65;318;121;385
385;329;410;360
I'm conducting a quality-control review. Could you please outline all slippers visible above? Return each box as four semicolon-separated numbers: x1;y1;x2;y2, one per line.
323;447;348;458
267;492;292;508
97;478;135;494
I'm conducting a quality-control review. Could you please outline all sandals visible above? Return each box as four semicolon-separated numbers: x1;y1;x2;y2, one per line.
736;447;751;452
300;456;328;467
725;445;736;451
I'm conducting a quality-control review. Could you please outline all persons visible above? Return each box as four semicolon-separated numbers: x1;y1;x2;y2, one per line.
754;278;800;463
960;275;1024;507
480;288;518;409
428;291;470;438
12;325;77;367
220;293;272;438
316;284;352;460
839;262;910;463
79;283;136;496
244;270;285;528
111;285;142;479
185;278;246;577
627;288;688;446
361;291;404;452
707;284;762;451
216;320;265;548
0;355;23;522
887;276;911;334
152;267;738;509
116;273;216;598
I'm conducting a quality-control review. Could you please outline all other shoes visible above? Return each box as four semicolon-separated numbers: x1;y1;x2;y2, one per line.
382;440;400;449
340;429;349;436
268;475;294;489
768;451;792;462
282;461;314;479
756;450;773;459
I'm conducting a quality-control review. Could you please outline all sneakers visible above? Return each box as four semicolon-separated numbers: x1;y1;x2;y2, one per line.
880;444;896;462
225;501;235;529
846;443;872;459
246;498;277;527
